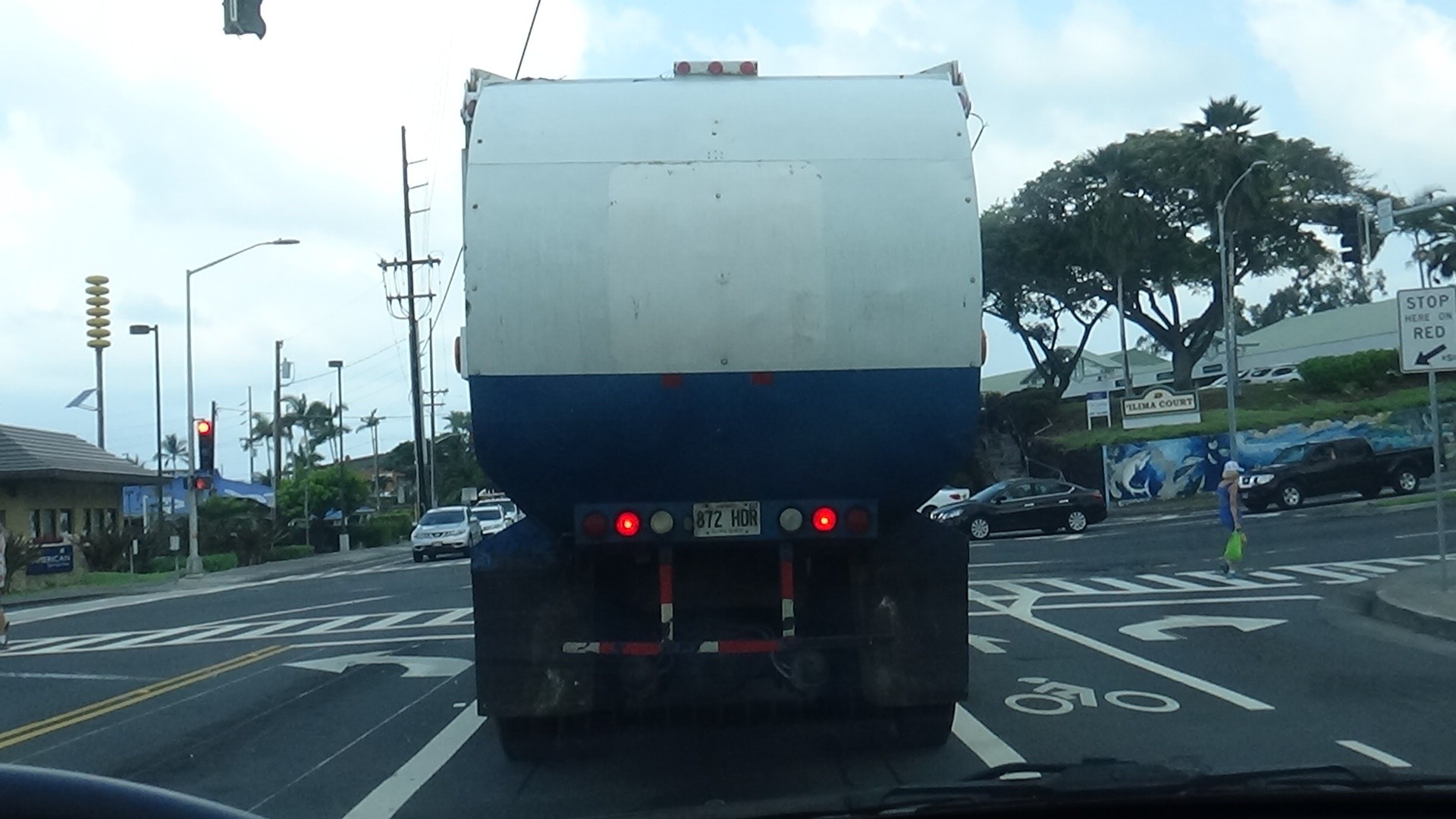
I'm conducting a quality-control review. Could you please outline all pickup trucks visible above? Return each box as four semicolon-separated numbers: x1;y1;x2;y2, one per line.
1237;435;1435;514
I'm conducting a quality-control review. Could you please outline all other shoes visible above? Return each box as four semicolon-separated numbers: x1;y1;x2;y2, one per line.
1220;562;1233;578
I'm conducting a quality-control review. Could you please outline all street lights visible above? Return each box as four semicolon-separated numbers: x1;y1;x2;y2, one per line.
1217;159;1268;512
85;275;111;451
184;239;299;578
129;324;165;521
328;361;343;534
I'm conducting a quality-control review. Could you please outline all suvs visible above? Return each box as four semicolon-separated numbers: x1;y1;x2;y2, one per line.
410;505;484;562
475;498;527;522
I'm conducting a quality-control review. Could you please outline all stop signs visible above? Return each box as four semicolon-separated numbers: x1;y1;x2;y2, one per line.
1397;283;1455;374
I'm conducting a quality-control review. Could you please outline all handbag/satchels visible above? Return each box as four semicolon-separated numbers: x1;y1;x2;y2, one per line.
1224;528;1243;563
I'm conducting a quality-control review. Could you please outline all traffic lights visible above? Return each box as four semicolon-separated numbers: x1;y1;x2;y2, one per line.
1339;206;1363;264
197;421;214;470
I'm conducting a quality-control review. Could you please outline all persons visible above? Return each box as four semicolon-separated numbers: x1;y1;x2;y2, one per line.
1217;457;1248;579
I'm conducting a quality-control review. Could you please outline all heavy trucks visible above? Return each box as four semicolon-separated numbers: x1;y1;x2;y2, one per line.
454;61;982;756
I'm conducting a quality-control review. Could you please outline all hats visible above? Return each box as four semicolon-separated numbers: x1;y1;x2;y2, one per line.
1223;461;1244;472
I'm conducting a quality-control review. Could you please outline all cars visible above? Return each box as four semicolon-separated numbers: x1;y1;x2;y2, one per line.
917;483;970;520
1197;366;1303;391
470;504;512;535
930;475;1109;541
